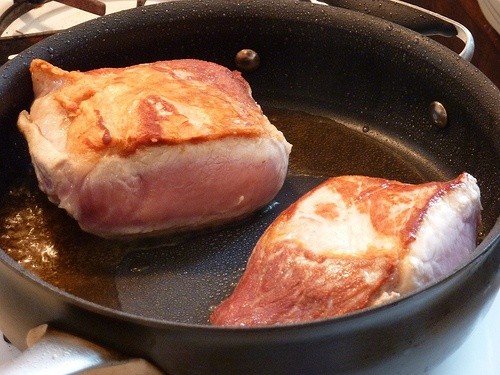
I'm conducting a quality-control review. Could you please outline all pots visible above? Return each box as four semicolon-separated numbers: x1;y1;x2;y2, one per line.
0;0;500;374
